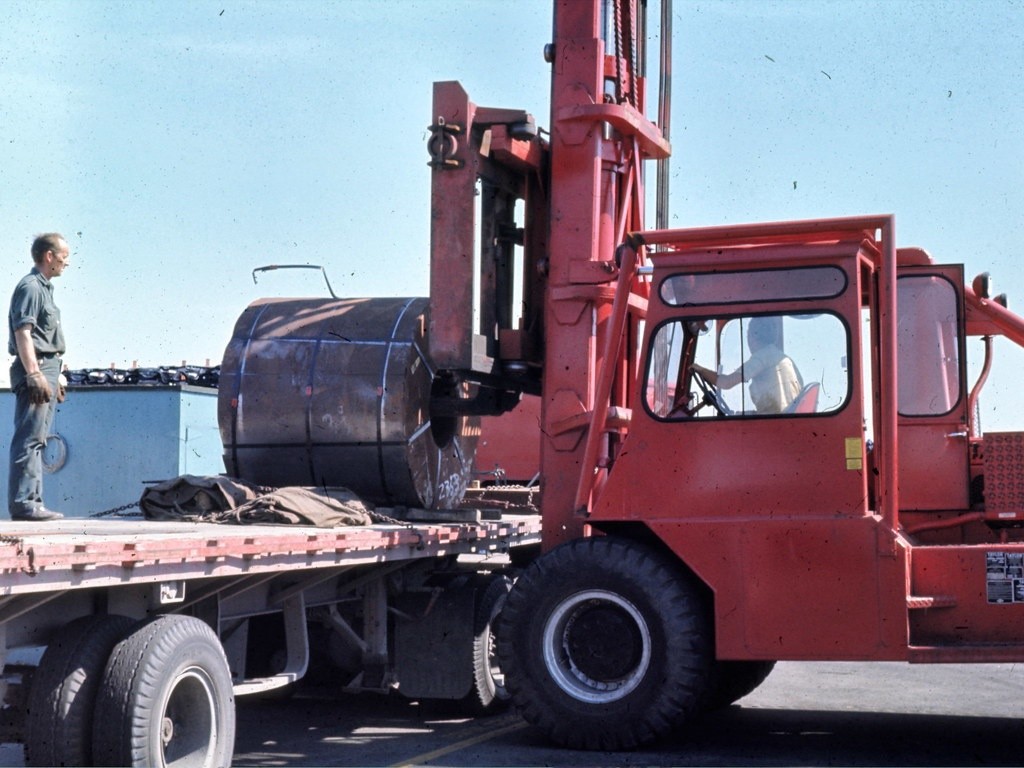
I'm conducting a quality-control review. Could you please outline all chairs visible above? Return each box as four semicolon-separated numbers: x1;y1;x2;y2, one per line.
786;382;820;412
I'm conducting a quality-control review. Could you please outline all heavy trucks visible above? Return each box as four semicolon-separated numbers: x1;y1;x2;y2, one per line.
0;339;686;768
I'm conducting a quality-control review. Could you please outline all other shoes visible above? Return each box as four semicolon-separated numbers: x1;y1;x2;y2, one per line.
11;507;64;521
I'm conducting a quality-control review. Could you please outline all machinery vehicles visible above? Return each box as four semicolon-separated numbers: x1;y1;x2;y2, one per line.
488;1;1023;768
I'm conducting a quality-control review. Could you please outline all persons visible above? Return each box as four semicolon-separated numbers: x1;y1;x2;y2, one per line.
688;318;803;414
8;233;69;521
